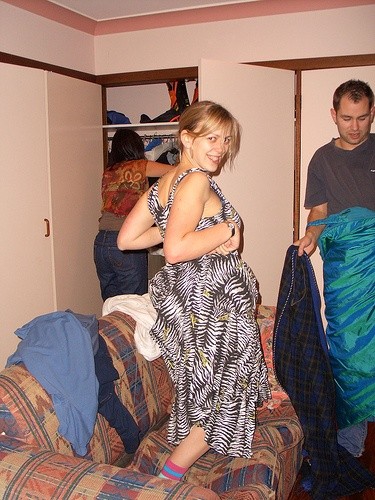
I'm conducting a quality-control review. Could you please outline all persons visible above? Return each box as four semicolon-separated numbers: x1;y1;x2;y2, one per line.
94;129;177;303
293;80;375;457
117;100;271;483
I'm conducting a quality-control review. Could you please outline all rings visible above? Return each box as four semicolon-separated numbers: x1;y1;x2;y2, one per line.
229;251;231;254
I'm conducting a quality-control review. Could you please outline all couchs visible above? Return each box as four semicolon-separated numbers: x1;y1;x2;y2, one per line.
0;305;306;500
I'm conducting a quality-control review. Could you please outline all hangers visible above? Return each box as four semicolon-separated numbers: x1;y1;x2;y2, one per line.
107;133;179;154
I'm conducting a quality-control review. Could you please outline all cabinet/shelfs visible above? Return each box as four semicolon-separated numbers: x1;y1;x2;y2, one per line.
104;58;375;349
0;61;103;373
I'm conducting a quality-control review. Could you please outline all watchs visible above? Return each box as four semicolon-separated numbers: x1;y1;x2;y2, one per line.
223;221;235;237
